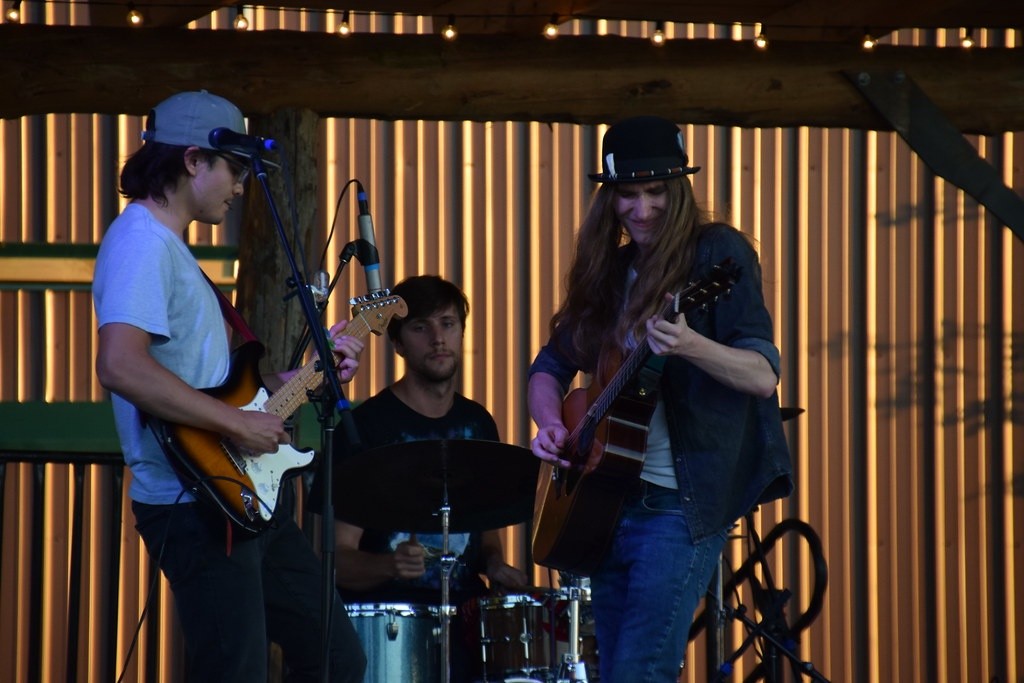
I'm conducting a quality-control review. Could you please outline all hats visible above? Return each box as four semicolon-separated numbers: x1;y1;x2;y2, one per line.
140;88;279;168
588;115;700;184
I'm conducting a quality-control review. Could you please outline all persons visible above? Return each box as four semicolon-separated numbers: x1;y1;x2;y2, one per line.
521;114;791;683
329;274;531;682
87;89;368;682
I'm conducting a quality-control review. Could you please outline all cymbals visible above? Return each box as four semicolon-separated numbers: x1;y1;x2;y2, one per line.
356;438;539;535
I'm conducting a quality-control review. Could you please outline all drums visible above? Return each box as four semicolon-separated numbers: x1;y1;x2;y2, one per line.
343;601;442;682
479;589;585;683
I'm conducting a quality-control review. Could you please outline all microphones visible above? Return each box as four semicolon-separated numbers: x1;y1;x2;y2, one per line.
208;126;284;153
356;183;384;297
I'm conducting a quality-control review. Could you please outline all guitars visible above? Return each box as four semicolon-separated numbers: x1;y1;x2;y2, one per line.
531;266;739;570
151;289;408;538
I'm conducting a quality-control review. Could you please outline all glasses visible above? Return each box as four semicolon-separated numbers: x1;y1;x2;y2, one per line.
199;148;250;183
